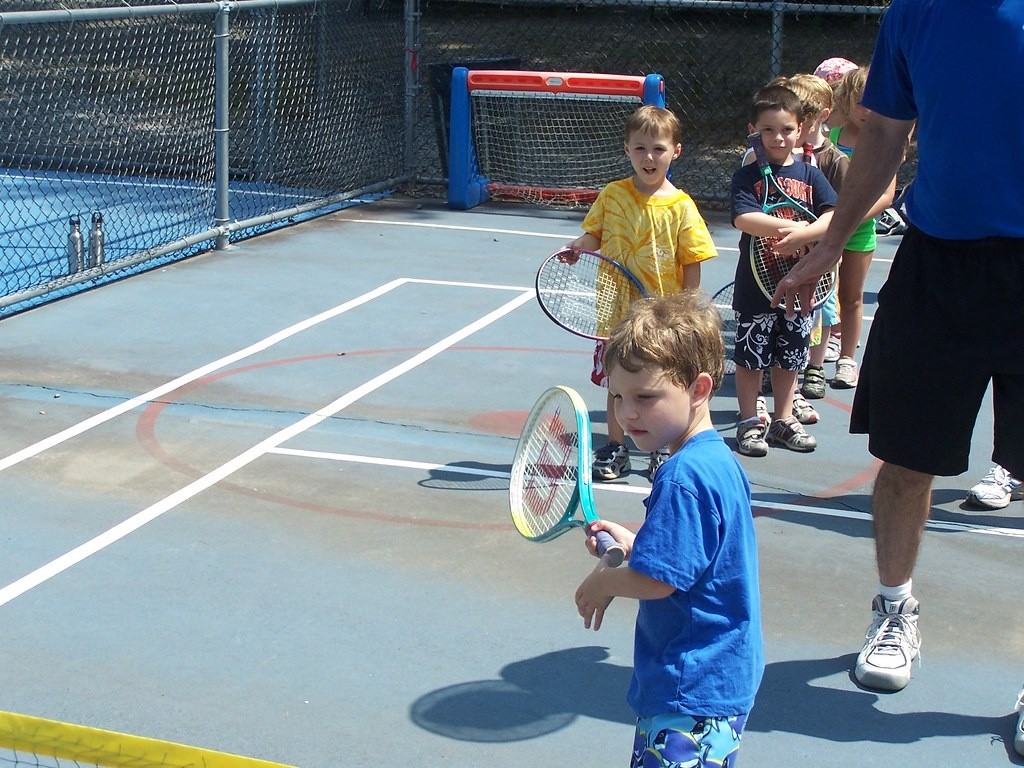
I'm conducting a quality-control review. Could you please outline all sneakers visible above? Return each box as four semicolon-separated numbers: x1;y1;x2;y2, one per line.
792;388;819;423
824;335;841;361
1013;689;1024;757
801;364;826;398
767;414;817;451
592;441;631;479
648;448;670;480
831;356;858;388
966;465;1024;510
798;349;810;373
735;416;768;457
855;593;922;690
761;368;772;394
736;395;771;424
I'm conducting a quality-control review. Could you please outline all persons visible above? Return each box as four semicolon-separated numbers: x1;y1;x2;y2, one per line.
772;1;1024;756
556;105;719;484
730;56;897;457
575;288;765;768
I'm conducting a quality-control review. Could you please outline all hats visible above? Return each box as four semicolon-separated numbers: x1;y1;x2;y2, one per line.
813;58;858;87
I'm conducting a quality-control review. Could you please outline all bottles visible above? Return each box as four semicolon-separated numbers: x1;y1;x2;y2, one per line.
89;211;104;267
68;214;84;274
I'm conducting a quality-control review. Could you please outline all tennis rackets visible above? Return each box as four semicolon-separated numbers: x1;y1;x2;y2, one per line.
745;131;840;313
712;279;738;377
535;248;650;342
506;385;627;570
